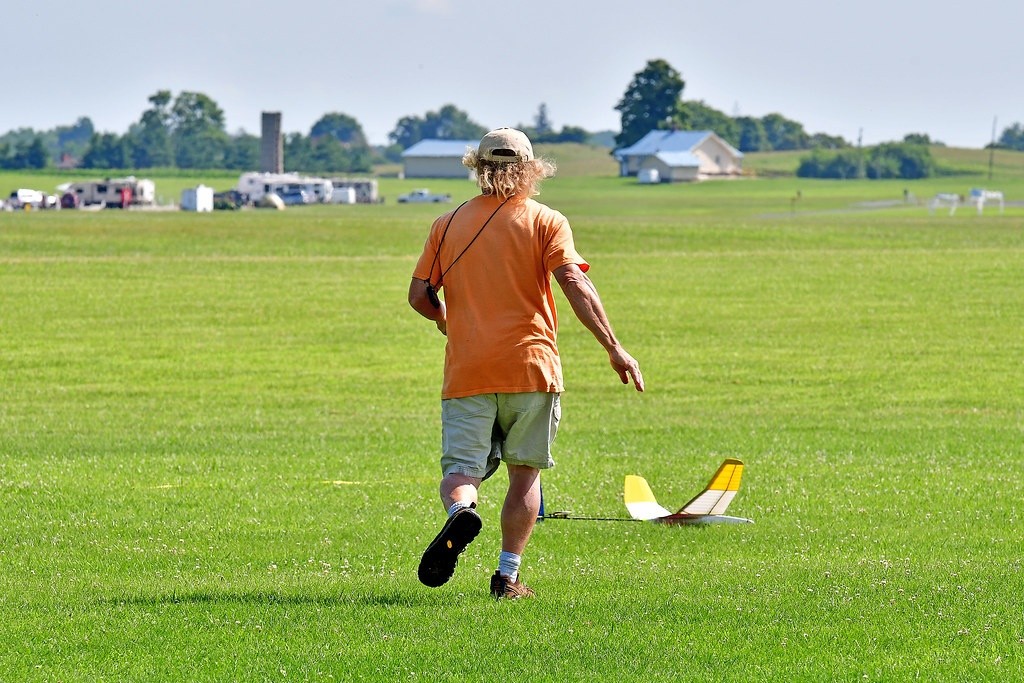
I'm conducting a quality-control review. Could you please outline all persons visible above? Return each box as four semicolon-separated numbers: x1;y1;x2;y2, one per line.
407;129;645;600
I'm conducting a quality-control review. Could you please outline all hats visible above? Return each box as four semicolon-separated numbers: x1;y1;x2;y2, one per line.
478;127;533;163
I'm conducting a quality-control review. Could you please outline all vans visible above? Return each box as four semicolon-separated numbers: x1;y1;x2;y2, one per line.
277;188;307;203
8;190;46;208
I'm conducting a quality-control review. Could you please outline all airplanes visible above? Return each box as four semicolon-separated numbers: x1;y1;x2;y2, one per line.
623;458;754;527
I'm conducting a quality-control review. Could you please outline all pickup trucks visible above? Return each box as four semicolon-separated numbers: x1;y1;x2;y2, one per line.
399;189;452;201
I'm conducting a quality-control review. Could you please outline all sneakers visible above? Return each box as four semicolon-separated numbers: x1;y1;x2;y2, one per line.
489;569;535;597
418;502;483;588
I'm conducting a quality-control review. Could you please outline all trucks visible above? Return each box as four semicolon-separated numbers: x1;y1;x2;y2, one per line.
68;177;153;206
262;179;332;205
